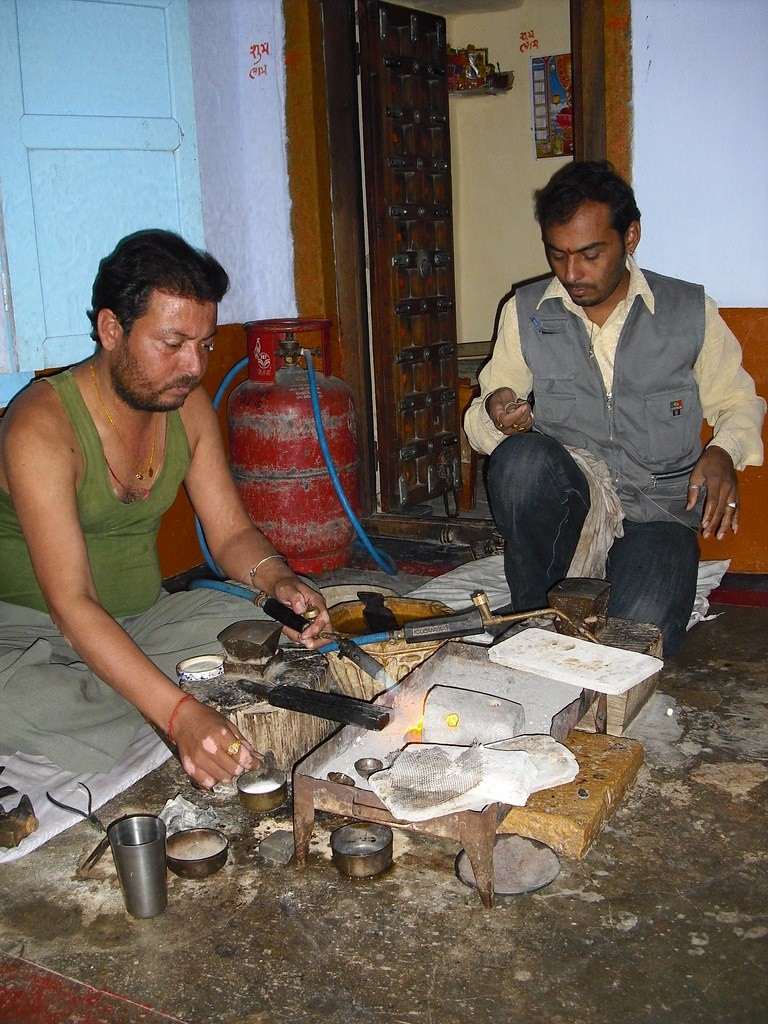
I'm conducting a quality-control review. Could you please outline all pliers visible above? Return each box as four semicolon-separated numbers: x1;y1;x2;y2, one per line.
46;781;107;834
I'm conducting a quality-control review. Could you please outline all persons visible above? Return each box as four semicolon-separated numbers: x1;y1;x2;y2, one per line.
463;160;766;658
0;227;334;789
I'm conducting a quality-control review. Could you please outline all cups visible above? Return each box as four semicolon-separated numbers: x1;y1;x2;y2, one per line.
327;772;355;786
355;758;383;778
108;817;168;919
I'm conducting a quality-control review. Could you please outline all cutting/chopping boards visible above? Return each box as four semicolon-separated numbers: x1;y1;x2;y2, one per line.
486;627;664;695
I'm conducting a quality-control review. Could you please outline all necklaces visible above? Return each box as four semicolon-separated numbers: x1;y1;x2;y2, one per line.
91;357;158;479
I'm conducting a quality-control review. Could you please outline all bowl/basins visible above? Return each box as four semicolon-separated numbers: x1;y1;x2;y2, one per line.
164;828;229;879
329;822;394;877
236;767;288;813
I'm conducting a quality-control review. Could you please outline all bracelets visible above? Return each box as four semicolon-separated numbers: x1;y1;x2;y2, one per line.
168;694;195;746
249;555;287;590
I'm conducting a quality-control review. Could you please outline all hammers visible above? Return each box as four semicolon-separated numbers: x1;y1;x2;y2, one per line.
0;794;39;849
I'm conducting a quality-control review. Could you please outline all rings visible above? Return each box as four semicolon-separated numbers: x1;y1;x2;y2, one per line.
689;484;701;492
726;502;737;509
517;426;524;432
512;423;518;430
227;741;241;756
498;417;503;428
